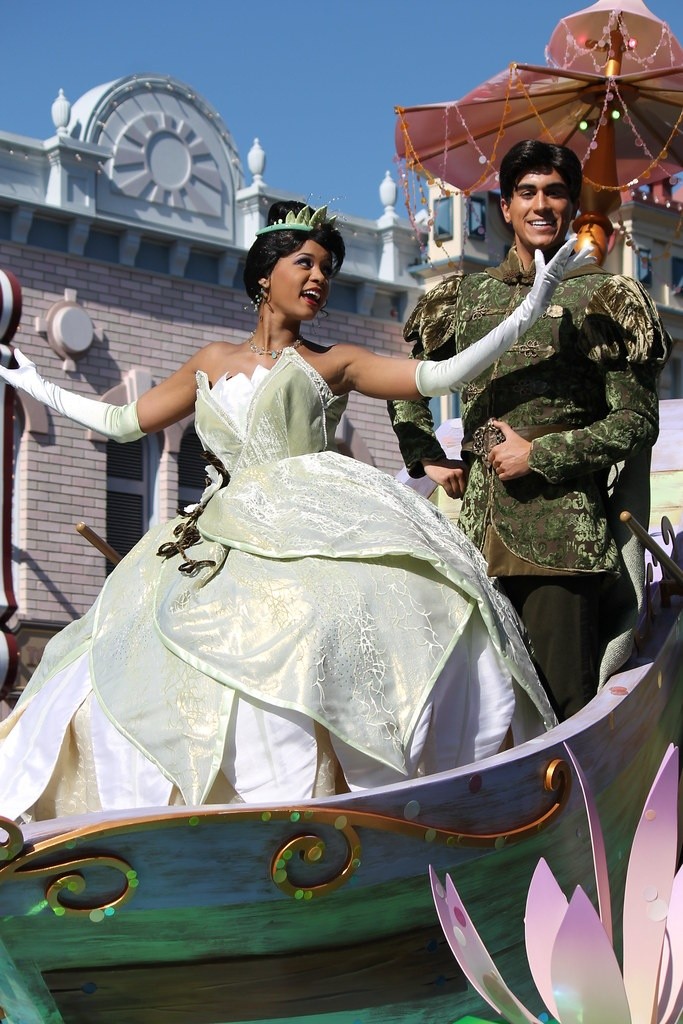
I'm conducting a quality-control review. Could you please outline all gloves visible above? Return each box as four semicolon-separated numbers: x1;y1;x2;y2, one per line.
415;230;598;398
0;348;147;445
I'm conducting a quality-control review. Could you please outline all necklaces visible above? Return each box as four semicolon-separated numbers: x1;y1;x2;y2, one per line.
247;329;304;359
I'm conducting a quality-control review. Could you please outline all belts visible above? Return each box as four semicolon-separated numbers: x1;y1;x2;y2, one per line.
461;424;575;471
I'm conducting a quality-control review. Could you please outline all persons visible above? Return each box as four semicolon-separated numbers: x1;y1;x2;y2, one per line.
386;141;675;724
0;200;598;823
